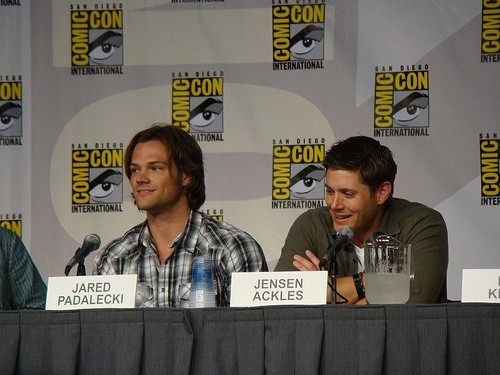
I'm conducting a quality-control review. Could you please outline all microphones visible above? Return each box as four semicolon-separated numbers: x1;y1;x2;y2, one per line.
319;225;355;268
65;233;101;275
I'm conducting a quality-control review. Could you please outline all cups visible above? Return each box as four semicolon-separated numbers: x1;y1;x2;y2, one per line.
364;236;410;306
188;256;216;309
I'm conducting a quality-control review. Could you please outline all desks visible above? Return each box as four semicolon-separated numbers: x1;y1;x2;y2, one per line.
1;303;500;375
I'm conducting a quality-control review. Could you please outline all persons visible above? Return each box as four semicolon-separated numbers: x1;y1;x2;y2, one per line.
92;124;268;309
0;224;47;312
273;136;449;303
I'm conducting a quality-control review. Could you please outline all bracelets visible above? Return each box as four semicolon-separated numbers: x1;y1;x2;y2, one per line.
353;273;366;299
